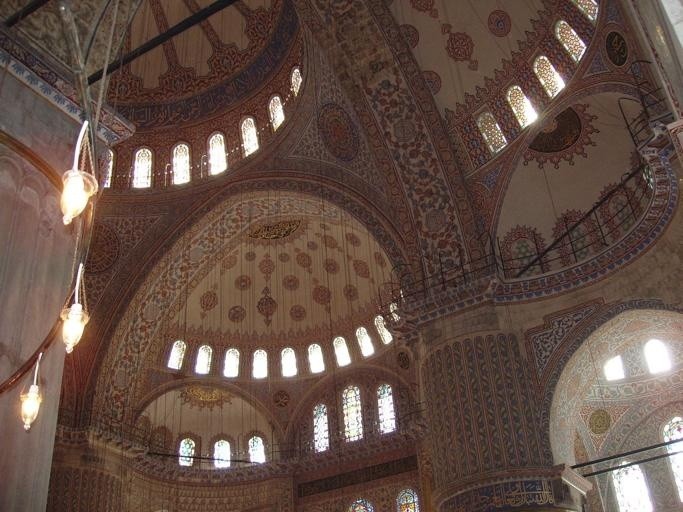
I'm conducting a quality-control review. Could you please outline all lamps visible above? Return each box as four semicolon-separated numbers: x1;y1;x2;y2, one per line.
19;118;96;431
